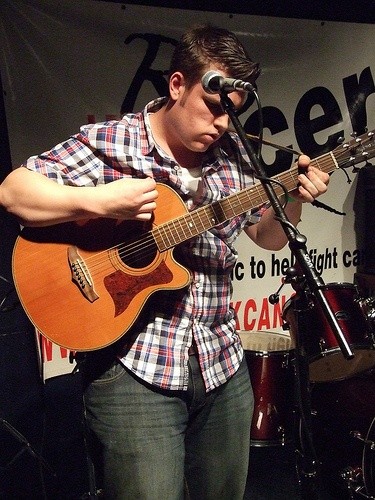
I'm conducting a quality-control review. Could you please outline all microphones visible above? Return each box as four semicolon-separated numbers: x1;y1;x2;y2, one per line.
201;69;256;94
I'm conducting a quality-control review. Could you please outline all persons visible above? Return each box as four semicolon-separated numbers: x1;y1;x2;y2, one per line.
0;23;330;500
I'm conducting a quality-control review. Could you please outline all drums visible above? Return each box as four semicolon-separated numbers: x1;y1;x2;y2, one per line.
302;377;375;500
281;282;375;384
235;329;296;447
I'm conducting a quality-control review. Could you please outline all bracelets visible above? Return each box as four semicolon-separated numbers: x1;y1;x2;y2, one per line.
281;196;295;203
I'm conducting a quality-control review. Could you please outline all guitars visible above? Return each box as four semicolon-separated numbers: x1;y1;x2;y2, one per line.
11;127;375;352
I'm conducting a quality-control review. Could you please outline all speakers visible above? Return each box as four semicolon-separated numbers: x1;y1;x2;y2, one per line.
0;272;115;499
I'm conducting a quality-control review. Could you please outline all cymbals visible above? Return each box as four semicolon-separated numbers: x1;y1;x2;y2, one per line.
228;129;304;156
312;200;346;216
